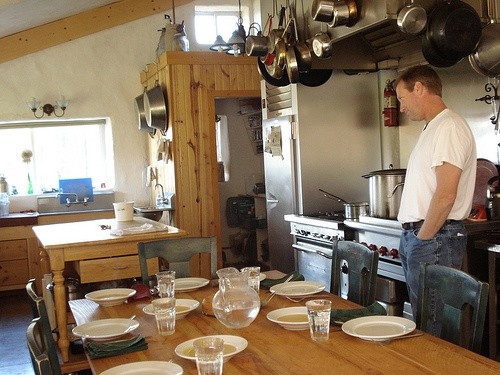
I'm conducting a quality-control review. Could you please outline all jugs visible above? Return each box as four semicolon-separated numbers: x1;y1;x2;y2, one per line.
212;266;261;328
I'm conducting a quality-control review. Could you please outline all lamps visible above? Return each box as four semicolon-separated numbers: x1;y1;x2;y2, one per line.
209;0;262;58
26;95;70;119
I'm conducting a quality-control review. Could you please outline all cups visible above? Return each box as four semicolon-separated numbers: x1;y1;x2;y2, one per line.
240;266;260;295
305;300;332;343
156;271;175;298
151;298;177;336
193;336;224;375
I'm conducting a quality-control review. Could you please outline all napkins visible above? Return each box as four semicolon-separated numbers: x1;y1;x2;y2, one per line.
259;272;304;290
331;302;388;323
87;335;147;358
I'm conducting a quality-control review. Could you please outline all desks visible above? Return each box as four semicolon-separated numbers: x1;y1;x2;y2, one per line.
31;215;191;362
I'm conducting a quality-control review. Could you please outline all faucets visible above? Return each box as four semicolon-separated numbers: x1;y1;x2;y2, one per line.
83;197;89;206
154;183;164;199
65;197;71;207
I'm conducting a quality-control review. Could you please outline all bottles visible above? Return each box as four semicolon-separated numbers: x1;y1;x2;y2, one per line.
0;177;10;216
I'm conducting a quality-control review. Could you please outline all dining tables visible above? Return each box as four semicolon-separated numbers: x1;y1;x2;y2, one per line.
69;270;500;375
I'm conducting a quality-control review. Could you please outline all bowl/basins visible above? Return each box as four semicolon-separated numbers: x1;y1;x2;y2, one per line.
112;201;134;221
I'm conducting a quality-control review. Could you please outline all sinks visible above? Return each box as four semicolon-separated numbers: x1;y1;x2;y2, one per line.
36;209;114;225
133;206;171;222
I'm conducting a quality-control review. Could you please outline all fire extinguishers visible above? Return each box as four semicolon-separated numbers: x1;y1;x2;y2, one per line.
382;79;399;126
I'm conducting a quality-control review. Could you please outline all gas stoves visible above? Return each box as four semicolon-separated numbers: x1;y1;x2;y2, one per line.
283;209;348;229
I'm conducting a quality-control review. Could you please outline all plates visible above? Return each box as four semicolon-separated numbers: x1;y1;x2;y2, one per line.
85;288;138;306
97;361;183;374
173;334;248;362
141;298;199;320
266;307;310;330
173;278;210;292
342;316;416;341
270;281;326;297
466;217;487;222
472;158;498;206
71;319;139;341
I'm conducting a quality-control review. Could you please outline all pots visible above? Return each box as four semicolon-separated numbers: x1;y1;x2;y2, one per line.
133;87;155;139
243;0;499;87
323;194;370;217
143;79;166;135
360;164;408;218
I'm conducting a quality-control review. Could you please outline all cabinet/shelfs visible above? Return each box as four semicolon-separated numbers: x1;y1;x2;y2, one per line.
0;226;40;291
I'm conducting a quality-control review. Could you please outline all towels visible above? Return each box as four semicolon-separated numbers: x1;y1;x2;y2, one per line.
328;301;388;326
111;223;169;236
127;284;151;302
155;137;170;164
260;271;304;290
146;166;156;187
87;335;148;359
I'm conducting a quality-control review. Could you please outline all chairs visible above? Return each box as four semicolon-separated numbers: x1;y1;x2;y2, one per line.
416;261;490;354
136;236;218;288
330;240;379;307
25;277;91;375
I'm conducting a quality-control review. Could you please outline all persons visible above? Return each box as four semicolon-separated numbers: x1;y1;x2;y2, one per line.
392;65;477;346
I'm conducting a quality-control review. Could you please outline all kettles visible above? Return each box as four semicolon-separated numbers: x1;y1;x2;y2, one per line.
485;174;500;221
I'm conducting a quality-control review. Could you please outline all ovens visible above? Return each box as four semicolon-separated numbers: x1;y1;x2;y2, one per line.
354;230;414;322
289;221;355;300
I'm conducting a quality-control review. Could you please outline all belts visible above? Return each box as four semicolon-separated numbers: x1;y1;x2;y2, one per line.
402;219;461;230
